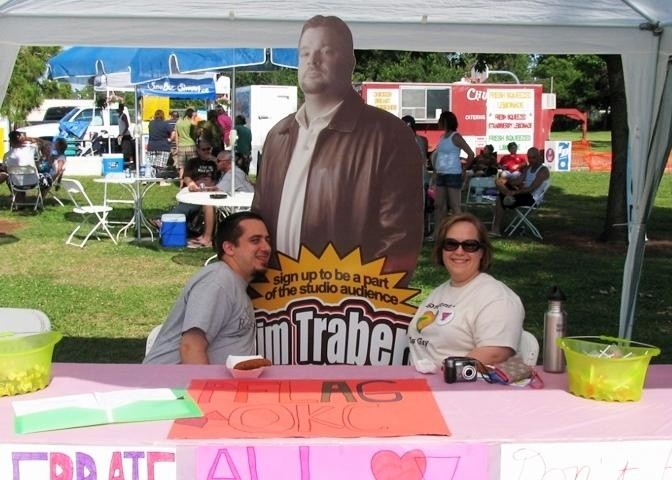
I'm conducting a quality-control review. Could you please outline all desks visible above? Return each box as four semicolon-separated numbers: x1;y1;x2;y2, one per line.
0;361;672;480
90;176;183;241
175;184;255;267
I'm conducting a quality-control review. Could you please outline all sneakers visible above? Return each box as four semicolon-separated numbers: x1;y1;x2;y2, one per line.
147;218;161;232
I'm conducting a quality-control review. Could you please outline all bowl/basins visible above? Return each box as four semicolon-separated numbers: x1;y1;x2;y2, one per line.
555;338;661;402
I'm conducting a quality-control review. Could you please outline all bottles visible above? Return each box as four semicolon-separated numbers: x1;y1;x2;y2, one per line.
475;183;483;203
123;158;152;178
541;285;568;373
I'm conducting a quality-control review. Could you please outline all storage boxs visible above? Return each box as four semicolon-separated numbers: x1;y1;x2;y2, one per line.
102;150;124;175
64;155;103;175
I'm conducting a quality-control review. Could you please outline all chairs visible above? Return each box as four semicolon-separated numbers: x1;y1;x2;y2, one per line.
61;178;118;249
145;322;162;357
0;164;66;215
102;172;139;231
515;330;539;366
424;167;550;240
0;307;50;341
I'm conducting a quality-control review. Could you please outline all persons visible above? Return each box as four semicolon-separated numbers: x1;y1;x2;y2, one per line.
401;110;551;244
140;211;272;365
247;15;424;298
406;213;526;375
1;105;257;249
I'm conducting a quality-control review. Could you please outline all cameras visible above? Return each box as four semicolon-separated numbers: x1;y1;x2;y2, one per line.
445;356;478;383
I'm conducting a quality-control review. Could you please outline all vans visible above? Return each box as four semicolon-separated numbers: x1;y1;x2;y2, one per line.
25;99;122;125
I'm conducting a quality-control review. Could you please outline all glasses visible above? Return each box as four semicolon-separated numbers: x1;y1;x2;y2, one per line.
442;238;483;253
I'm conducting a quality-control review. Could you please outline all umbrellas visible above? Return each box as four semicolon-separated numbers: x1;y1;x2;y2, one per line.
38;46;299;196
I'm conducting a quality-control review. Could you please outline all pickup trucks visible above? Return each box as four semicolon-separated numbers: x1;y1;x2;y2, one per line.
14;104;136;143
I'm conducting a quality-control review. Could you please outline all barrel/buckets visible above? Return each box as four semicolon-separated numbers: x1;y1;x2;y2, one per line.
557;327;661;401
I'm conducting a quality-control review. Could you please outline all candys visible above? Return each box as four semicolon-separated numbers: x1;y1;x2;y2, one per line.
586;345;649;401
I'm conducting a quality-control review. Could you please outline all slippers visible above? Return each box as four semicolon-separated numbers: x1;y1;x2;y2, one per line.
487;231;501;237
503;196;515;205
187;235;213;249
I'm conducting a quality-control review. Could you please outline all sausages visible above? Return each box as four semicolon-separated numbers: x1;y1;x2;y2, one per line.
234;359;272;370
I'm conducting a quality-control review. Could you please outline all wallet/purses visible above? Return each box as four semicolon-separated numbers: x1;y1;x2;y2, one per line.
493;361;545;389
